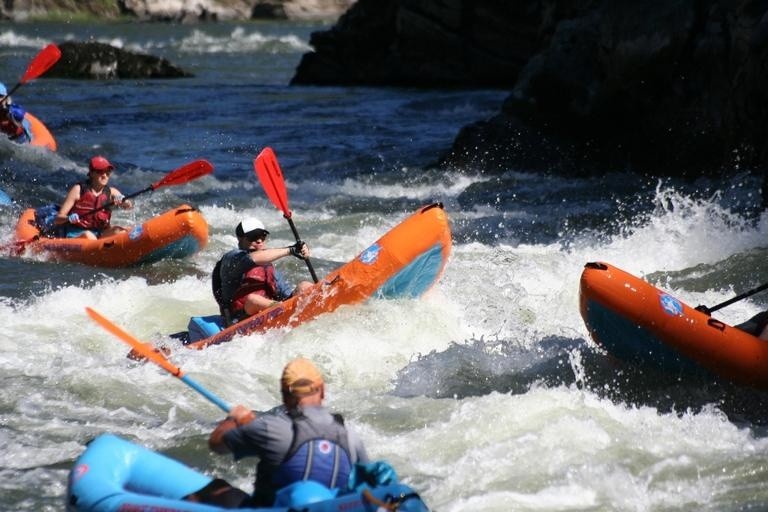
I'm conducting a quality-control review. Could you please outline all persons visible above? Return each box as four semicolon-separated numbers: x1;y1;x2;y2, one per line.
181;359;370;508
53;155;132;239
210;217;315;324
0;83;33;146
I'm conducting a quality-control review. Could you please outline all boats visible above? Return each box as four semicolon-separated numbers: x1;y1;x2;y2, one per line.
578;261;768;389
158;195;454;356
15;111;57;152
12;202;209;273
66;432;430;512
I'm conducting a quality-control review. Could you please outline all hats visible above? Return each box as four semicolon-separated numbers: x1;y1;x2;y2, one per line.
236;218;270;237
0;83;7;96
89;156;114;170
282;360;322;392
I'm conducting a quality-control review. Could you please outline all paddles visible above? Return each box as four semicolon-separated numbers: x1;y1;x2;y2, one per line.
695;282;768;317
254;147;319;285
0;159;214;257
85;307;233;414
0;43;63;104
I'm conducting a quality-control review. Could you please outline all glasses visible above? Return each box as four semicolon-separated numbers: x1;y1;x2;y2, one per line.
247;233;267;242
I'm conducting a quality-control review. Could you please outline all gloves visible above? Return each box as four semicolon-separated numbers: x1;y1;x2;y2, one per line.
68;213;80;224
